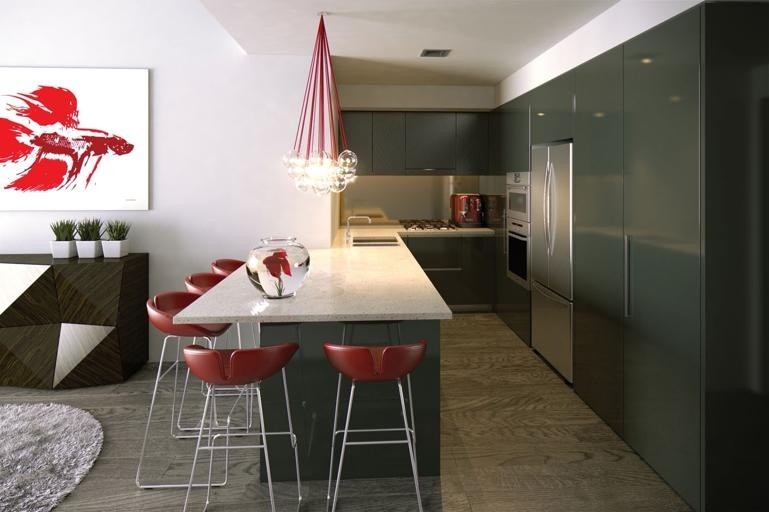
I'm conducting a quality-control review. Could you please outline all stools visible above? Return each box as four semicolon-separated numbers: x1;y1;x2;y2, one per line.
320;339;430;512
181;340;304;511
133;257;260;484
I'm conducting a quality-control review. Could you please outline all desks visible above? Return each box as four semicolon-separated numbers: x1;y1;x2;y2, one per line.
173;244;453;493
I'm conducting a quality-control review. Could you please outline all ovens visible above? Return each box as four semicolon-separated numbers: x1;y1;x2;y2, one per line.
505;185;530;289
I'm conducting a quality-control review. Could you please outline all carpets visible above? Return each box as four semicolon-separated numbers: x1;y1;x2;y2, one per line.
0;399;106;512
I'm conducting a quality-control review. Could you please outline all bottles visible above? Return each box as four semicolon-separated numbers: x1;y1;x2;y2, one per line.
247;237;311;300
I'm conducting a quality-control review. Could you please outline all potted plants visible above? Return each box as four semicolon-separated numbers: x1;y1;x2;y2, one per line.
75;215;105;258
101;219;132;259
49;216;78;258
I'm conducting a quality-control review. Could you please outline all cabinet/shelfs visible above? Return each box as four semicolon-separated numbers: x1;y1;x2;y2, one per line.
401;237;498;312
494;93;531;170
457;112;503;176
572;1;769;511
404;111;456;175
531;69;572;147
341;111;404;175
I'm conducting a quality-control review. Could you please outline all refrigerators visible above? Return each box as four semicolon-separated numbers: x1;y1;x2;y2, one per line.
531;143;574;383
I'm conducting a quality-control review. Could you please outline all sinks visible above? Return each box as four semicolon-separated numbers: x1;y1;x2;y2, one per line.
352;243;402;247
351;235;400;243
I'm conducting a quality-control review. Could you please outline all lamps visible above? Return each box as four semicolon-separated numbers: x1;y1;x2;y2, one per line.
278;11;359;195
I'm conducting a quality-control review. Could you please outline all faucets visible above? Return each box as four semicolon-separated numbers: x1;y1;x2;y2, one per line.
346;215;371;242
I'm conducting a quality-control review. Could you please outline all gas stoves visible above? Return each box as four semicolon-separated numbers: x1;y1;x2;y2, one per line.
403;218;455;230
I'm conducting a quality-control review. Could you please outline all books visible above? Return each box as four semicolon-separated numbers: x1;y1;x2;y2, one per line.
352;234;400;246
